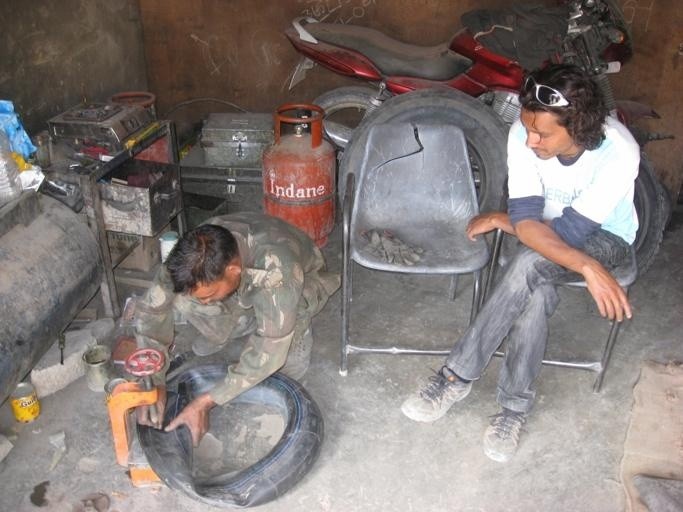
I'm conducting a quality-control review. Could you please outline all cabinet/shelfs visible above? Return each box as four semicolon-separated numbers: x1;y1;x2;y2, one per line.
24;120;188;321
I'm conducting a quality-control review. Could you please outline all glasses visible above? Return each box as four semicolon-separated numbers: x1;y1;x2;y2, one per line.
522;73;573;107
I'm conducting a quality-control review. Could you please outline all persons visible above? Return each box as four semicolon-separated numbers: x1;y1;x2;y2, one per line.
134;211;342;449
401;60;641;462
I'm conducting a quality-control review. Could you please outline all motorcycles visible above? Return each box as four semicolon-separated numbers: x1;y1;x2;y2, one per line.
284;0;669;276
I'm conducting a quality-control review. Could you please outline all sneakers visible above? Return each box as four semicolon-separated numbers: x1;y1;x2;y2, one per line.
191;315;258;357
281;319;312;379
399;368;475;422
481;410;525;462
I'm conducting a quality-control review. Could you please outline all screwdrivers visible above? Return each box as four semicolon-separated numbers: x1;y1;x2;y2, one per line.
58;334;65;365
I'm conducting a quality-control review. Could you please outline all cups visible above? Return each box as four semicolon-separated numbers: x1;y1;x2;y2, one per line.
33;135;50;168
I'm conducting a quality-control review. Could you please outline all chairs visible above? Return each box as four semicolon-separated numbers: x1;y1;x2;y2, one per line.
336;119;491;379
481;171;638;394
